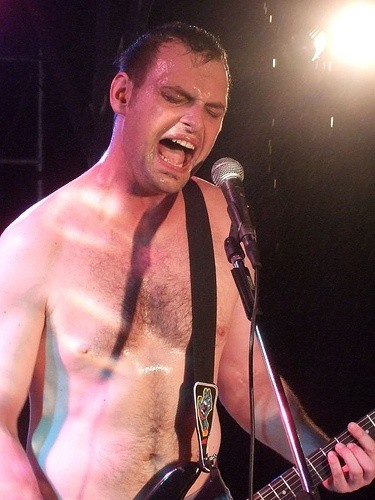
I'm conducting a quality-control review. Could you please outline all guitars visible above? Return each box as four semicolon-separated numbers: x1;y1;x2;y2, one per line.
133;410;375;500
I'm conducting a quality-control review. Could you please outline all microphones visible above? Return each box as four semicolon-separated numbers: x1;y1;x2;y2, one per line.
211;157;263;270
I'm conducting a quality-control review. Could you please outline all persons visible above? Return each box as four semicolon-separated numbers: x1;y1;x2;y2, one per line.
0;19;375;500
